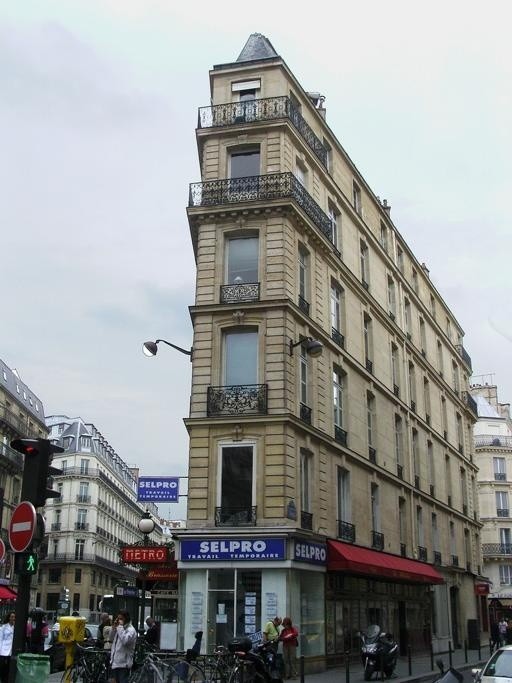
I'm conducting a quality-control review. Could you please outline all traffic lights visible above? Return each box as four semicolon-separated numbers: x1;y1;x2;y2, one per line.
23;552;38;576
10;437;64;507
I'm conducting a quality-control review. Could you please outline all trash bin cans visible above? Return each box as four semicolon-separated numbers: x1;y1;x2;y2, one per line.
15;653;51;683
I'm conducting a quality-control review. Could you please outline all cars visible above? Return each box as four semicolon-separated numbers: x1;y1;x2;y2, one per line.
301;620;326;649
471;645;512;683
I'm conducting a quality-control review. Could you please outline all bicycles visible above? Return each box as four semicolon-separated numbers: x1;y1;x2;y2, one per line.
60;636;240;683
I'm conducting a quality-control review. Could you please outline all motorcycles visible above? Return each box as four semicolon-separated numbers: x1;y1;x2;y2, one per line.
432;658;463;683
353;624;401;681
228;636;284;683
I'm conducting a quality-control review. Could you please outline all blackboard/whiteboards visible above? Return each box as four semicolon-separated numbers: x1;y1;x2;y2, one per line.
159;621;177;650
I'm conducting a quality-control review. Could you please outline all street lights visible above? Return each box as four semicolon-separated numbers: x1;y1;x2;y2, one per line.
138;508;155;635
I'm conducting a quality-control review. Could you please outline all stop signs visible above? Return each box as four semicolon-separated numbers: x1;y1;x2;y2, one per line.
8;501;37;553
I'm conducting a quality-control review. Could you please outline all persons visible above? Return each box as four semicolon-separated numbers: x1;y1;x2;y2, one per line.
491;618;512;651
0;609;160;683
263;617;299;680
